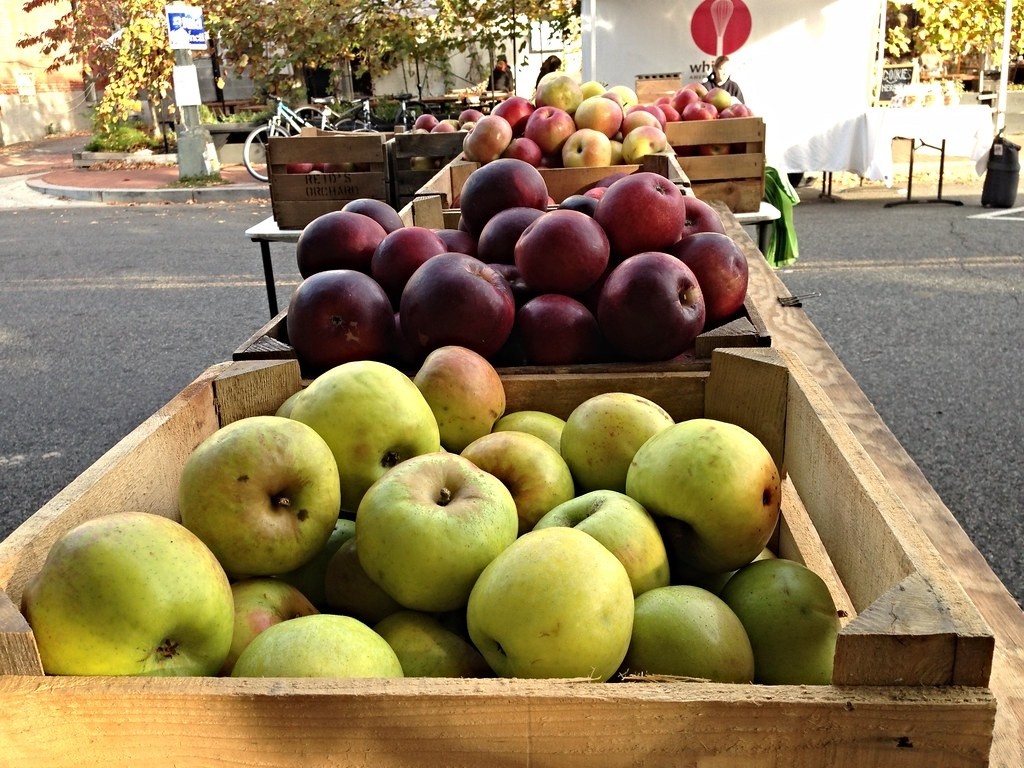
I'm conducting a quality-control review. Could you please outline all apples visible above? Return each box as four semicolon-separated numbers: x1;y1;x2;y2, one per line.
407;109;484;172
283;158;751;370
462;72;757;167
22;345;843;688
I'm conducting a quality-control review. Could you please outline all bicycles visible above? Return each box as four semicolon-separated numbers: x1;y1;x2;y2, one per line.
242;93;429;183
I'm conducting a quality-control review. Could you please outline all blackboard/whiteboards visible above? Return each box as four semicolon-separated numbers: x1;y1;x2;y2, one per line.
872;63;921;106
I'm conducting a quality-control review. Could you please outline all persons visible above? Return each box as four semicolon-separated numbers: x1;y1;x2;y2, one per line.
702;56;744;105
486;52;513;104
535;55;562;92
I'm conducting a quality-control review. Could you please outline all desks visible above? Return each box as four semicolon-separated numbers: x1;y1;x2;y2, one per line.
245;200;781;321
704;198;1024;768
817;104;995;208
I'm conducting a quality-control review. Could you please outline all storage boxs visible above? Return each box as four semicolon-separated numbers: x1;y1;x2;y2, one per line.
0;113;997;768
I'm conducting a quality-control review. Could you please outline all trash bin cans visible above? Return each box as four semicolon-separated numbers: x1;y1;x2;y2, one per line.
979;137;1022;207
